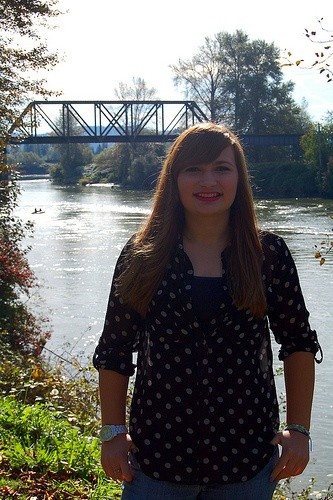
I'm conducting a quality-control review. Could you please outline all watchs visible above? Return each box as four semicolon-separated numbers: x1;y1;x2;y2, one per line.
99;424;128;443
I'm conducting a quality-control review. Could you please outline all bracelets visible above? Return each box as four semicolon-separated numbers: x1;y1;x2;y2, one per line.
282;424;314;452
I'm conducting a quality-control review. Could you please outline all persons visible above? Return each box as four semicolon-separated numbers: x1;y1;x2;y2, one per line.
91;120;323;500
